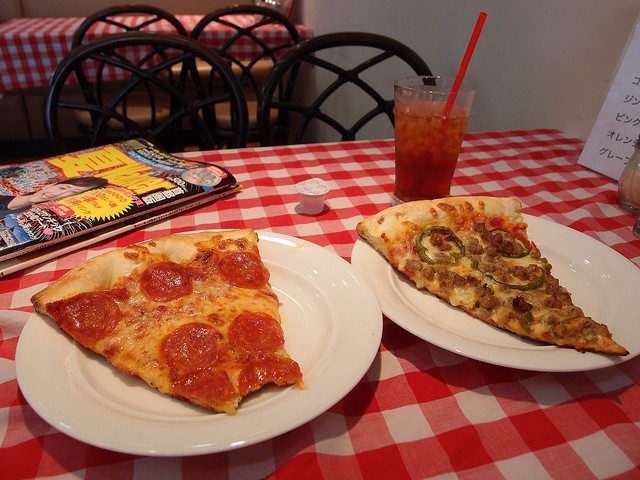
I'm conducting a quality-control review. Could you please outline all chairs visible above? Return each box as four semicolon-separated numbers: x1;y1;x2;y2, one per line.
72;5;190;131
190;6;299;146
256;31;436;145
45;32;250;148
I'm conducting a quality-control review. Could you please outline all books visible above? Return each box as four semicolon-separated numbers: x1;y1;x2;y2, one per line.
576;14;640;183
0;136;238;263
0;184;243;278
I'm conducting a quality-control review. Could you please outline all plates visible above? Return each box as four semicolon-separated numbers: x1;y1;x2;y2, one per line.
351;212;640;373
15;229;384;457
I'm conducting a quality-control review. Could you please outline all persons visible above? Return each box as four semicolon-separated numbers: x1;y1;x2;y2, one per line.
0;176;108;222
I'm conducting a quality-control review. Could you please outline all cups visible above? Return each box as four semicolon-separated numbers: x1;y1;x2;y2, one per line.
296;177;330;215
392;76;477;207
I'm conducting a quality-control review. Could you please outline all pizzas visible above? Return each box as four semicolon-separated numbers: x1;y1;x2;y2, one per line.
30;227;306;413
356;196;629;356
